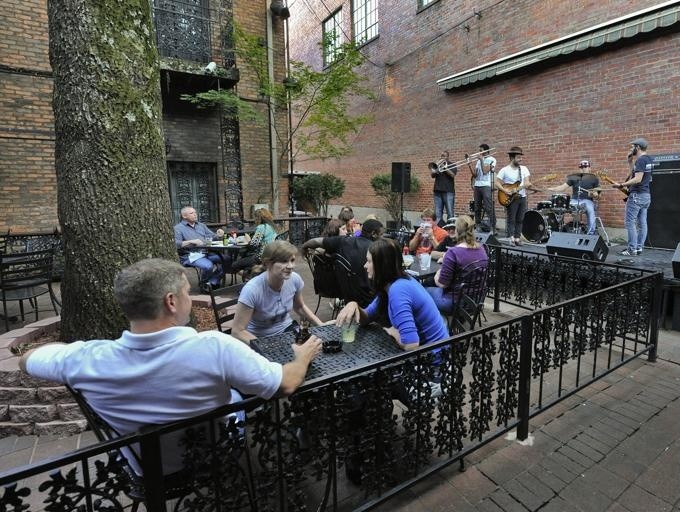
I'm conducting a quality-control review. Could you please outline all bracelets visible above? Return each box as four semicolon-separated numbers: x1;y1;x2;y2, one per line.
620;183;623;186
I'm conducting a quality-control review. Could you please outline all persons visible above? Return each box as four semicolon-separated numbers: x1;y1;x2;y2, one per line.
496;147;536;247
302;207;490;314
431;150;456;224
231;208;278;281
335;238;450;392
174;205;229;293
549;160;600;234
613;138;653;256
468;144;496;230
230;239;335;350
21;259;322;484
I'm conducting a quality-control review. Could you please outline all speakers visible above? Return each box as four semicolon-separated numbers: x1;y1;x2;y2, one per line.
672;242;680;278
643;171;680;250
472;233;502;245
391;161;411;193
545;231;608;266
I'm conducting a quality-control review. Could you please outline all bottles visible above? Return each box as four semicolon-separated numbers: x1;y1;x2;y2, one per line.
272;297;288;326
222;232;228;246
295;316;311;344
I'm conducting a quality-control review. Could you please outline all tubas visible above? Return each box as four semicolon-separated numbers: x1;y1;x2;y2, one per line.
428;158;448;174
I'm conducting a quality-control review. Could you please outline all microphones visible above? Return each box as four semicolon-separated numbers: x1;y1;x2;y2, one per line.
489;160;494;165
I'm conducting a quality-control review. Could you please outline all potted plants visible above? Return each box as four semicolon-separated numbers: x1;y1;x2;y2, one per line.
371;172;421;236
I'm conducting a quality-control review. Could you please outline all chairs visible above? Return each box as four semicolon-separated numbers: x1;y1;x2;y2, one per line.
2;248;59;332
178;222;295;294
448;283;483;365
1;224;64;311
65;384;253;512
210;284;248;335
298;224;494;328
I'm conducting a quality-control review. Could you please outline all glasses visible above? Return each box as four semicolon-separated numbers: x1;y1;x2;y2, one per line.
580;166;589;169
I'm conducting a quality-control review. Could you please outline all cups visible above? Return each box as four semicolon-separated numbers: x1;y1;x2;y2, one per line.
418;254;431;271
340;322;359;344
402;255;414;269
205;237;212;247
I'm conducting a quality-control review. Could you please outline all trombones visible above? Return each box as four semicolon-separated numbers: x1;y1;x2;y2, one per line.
439;147;497;173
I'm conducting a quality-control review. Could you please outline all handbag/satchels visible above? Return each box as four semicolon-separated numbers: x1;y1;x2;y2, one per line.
240;246;260;258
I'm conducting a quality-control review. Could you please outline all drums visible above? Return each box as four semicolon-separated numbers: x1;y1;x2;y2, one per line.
521;193;573;243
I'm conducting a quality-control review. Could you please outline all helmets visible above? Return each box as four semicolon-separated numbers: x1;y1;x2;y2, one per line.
578;160;590;167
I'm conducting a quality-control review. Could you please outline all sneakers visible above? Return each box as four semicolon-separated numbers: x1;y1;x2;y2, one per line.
515;240;523;246
617;248;642;256
198;279;213;293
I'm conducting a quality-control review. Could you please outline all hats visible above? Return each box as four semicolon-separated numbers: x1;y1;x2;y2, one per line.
629;138;648;148
506;147;524;155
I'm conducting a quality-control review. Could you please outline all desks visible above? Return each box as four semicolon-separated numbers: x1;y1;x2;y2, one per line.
249;322;406;391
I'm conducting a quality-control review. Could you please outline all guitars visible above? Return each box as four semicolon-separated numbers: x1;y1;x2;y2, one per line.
595;168;630;202
498;174;555;206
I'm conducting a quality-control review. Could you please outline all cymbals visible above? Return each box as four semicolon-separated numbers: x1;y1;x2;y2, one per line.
566;172;599;189
